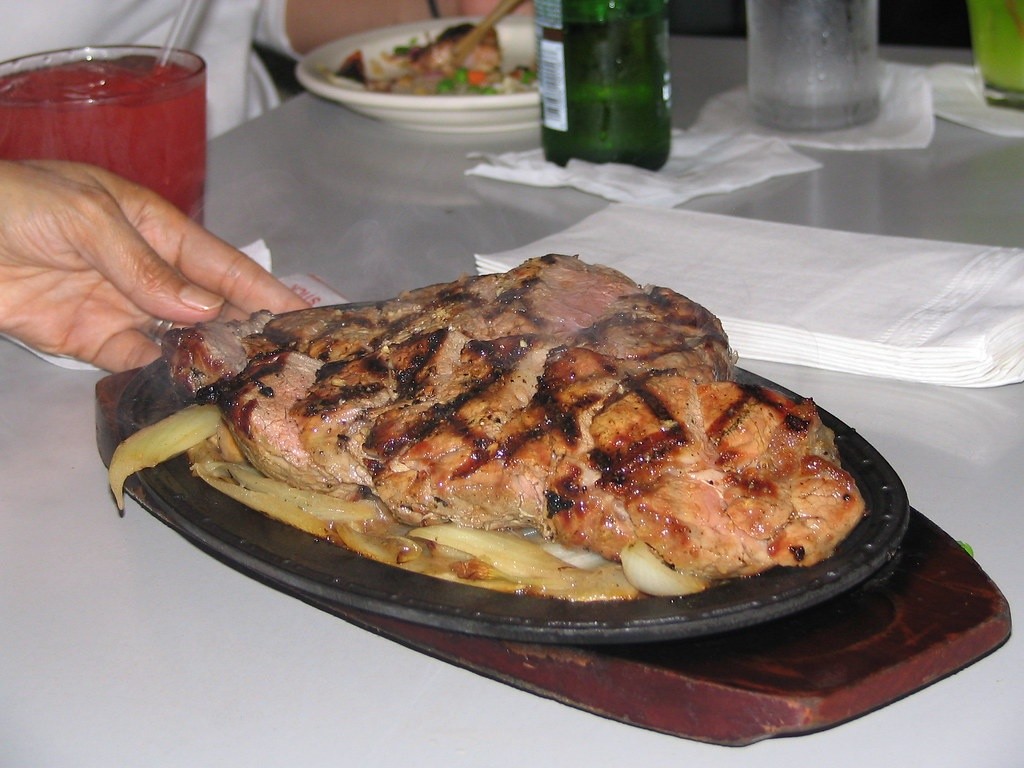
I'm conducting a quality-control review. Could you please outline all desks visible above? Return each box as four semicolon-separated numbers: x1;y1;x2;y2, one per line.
0;29;1024;768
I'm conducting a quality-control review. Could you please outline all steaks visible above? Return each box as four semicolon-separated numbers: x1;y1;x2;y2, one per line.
158;249;866;582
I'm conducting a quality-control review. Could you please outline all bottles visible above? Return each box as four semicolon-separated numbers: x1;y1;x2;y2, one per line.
531;0;670;174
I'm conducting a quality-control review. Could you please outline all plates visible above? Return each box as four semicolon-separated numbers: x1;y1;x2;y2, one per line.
113;285;909;644
294;15;643;132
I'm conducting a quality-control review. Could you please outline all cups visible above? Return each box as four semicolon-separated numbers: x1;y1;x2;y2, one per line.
0;40;209;336
967;0;1024;109
743;0;884;128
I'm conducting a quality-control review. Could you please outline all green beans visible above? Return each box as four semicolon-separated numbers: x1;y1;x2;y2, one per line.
435;65;537;97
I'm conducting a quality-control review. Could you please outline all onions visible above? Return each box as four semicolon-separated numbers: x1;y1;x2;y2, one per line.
109;404;706;601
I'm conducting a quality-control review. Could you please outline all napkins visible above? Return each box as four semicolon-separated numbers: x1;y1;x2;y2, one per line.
0;234;272;370
685;60;936;151
463;128;825;210
475;204;1024;388
923;61;1023;138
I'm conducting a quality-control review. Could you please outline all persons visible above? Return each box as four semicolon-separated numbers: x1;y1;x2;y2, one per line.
0;157;313;374
0;0;533;143
